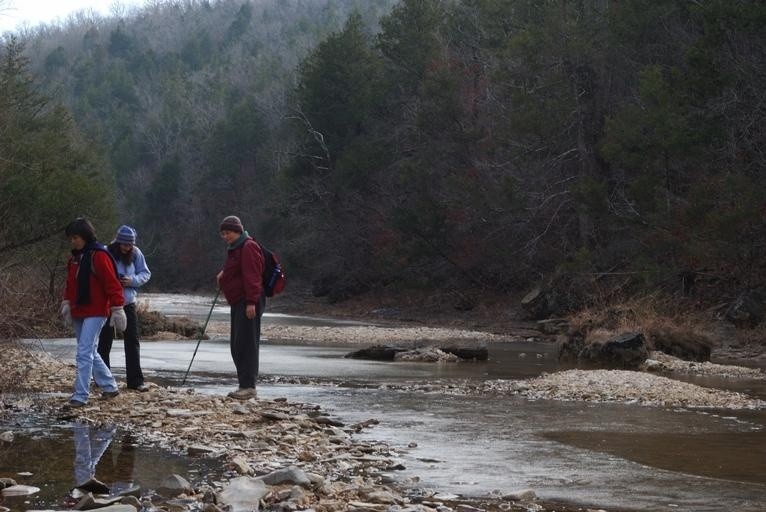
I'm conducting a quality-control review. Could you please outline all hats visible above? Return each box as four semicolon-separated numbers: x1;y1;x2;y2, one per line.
219;216;243;232
115;225;135;245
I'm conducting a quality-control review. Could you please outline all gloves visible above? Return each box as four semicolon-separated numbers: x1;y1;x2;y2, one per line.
109;306;127;331
61;300;72;326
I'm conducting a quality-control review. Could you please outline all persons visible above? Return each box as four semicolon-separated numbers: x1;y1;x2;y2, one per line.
97;224;151;395
215;215;267;400
64;418;115;494
61;218;128;407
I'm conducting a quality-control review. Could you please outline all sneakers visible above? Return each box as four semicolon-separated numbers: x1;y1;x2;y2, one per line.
229;387;257;400
137;384;149;392
97;390;119;400
66;400;85;408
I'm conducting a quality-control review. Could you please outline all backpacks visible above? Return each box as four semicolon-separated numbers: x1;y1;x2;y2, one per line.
253;237;287;298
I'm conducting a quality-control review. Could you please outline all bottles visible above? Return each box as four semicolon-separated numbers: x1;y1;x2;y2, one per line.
267;263;282;289
